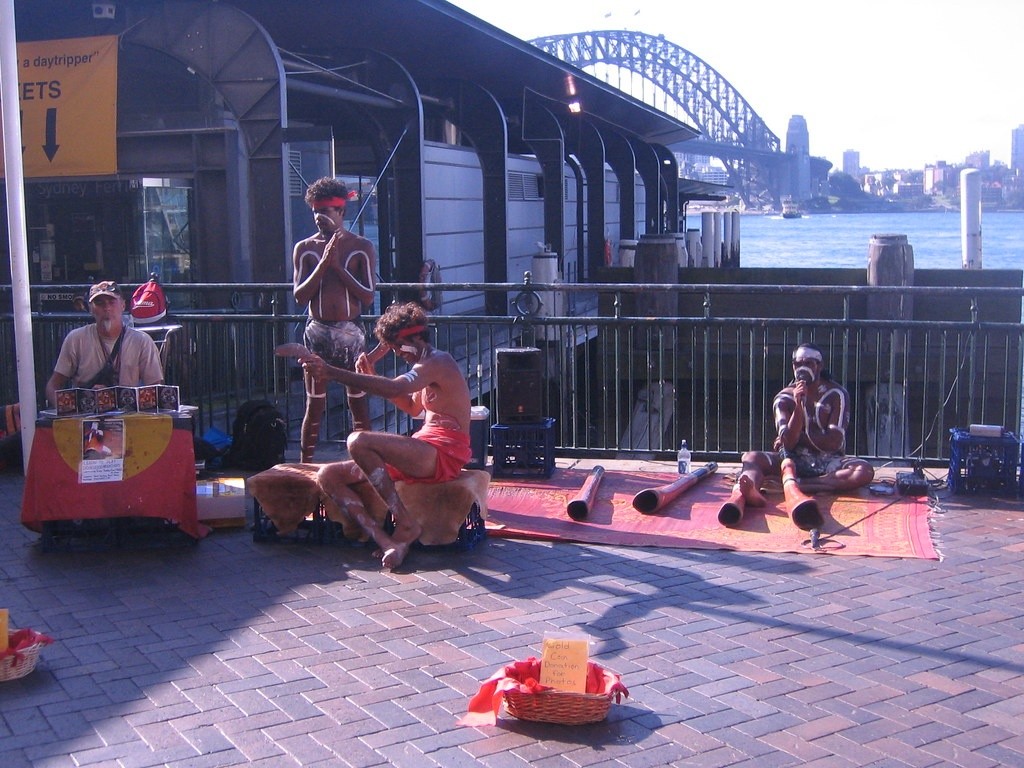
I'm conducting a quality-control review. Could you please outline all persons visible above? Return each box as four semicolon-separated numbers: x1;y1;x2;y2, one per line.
737;341;874;509
293;176;377;464
46;280;166;413
297;301;472;570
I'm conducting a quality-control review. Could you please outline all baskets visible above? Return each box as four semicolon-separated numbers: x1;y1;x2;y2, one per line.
503;662;620;725
0;628;42;682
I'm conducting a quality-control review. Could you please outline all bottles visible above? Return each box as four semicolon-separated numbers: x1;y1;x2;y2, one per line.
678;439;691;479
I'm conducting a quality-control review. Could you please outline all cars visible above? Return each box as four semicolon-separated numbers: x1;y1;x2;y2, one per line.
131;325;196;383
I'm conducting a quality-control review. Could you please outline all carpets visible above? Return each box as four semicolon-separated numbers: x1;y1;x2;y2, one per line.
483;466;948;564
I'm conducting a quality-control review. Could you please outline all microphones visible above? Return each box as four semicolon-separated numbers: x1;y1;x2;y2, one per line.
799;374;808;403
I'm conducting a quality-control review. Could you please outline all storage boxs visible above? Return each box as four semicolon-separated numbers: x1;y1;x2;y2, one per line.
948;426;1021;499
251;465;491;553
195;478;248;531
491;418;558;480
412;406;492;469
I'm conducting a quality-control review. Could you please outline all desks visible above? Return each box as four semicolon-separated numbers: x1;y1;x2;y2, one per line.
21;405;214;555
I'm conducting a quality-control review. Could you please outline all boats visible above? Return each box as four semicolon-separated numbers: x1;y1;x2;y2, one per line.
782;194;801;217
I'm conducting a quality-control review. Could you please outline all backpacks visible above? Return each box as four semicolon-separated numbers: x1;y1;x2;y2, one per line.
230;400;288;470
130;279;167;340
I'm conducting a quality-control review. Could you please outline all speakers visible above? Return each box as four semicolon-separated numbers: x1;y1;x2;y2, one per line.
495;346;542;424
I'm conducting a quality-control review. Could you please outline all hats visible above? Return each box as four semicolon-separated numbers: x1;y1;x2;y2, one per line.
89;281;123;302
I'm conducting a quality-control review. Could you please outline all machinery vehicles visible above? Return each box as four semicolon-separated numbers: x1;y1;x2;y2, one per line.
130;187;190;310
